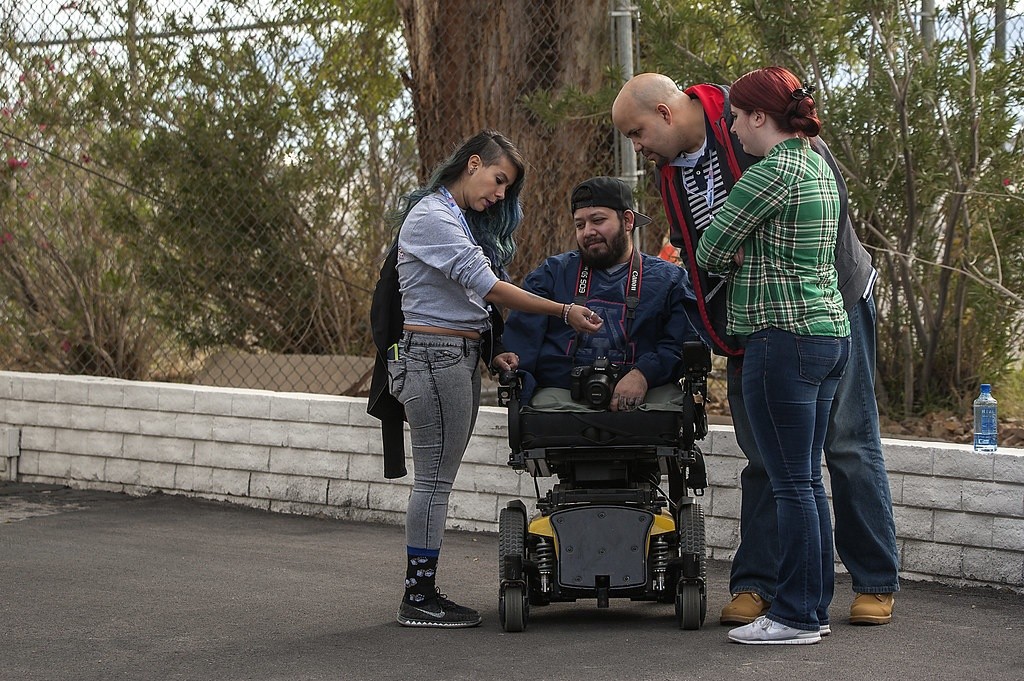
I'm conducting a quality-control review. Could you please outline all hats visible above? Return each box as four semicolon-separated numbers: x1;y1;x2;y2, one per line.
571;177;653;227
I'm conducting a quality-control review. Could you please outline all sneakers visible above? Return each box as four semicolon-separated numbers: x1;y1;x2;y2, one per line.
848;591;895;624
819;623;831;635
720;591;774;624
396;585;482;628
728;614;821;645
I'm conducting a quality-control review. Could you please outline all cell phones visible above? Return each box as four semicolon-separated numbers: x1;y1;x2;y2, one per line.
387;343;399;361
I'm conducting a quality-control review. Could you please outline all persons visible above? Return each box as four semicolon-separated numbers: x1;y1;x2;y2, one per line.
501;176;687;410
611;65;901;645
366;127;606;629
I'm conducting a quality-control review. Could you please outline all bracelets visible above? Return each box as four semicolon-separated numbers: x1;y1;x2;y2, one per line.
561;304;565;318
564;303;575;325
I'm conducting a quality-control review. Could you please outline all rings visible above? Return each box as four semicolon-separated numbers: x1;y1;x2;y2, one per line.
589;311;594;318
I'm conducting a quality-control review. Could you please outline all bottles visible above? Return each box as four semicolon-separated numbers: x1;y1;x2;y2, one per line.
974;383;998;453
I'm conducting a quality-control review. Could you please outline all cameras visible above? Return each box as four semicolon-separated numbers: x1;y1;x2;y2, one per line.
569;346;638;411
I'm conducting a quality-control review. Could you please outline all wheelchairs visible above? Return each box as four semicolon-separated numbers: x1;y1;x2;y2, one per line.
495;339;708;630
